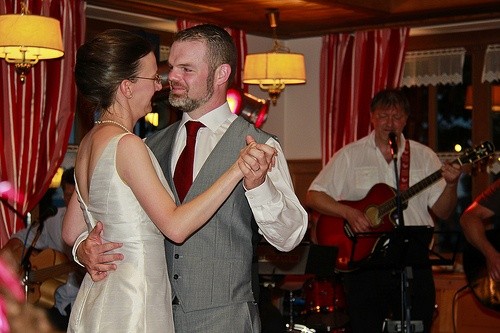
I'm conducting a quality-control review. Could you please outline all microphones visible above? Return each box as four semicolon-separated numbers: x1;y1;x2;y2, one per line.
388;131;398;159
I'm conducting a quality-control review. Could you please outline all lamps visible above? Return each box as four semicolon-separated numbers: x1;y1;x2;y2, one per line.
243;10;308;107
0;0;66;82
227;88;271;128
464;85;500;110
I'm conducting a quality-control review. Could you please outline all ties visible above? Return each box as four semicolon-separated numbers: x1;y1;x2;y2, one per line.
172;121;205;203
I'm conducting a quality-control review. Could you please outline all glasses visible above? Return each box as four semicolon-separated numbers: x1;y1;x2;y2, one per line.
113;72;163;94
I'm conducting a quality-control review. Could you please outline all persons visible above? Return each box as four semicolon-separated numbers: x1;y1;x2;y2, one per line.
459;179;500;283
0;166;88;333
71;24;307;333
305;89;462;333
61;27;278;333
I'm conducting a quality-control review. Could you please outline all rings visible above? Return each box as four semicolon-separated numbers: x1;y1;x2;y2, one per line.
256;167;260;172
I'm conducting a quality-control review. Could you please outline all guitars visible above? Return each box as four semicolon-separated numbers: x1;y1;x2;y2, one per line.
1;236;81;307
317;140;495;272
465;226;500;308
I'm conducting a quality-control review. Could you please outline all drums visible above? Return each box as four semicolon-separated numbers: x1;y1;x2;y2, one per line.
300;274;346;330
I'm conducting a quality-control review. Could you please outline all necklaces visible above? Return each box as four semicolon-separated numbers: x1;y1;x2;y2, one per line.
94;121;132;134
